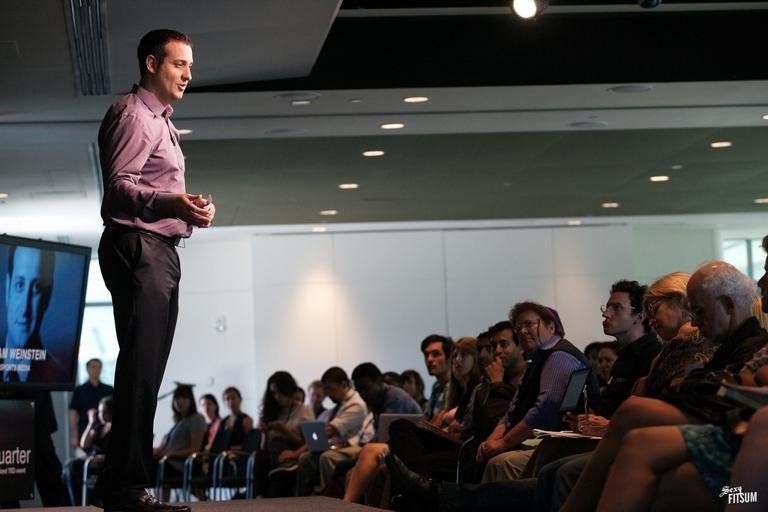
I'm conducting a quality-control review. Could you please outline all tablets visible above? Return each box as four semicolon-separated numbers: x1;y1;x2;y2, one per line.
557;367;594;415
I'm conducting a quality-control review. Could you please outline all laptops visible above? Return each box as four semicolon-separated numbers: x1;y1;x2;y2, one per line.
377;413;425;442
300;421;338;453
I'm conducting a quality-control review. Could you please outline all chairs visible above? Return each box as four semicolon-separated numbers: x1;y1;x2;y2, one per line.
81;449;258;508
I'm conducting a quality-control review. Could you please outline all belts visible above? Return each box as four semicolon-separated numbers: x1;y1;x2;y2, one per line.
130;226;182;247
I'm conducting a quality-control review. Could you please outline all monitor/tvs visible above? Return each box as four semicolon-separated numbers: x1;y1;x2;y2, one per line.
0;233;93;396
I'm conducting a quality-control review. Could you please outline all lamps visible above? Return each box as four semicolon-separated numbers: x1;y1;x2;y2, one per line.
508;0;548;20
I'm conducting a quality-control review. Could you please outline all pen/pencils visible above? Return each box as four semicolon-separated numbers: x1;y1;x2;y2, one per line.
583;390;588;414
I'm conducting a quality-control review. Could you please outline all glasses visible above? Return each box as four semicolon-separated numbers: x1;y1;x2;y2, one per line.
644;298;666;316
512;319;545;335
688;302;716;325
601;305;635;313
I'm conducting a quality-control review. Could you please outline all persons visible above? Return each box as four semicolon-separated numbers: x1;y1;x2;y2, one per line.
0;244;67;383
62;394;114;507
68;357;115;460
1;389;74;510
148;235;768;512
90;29;216;511
